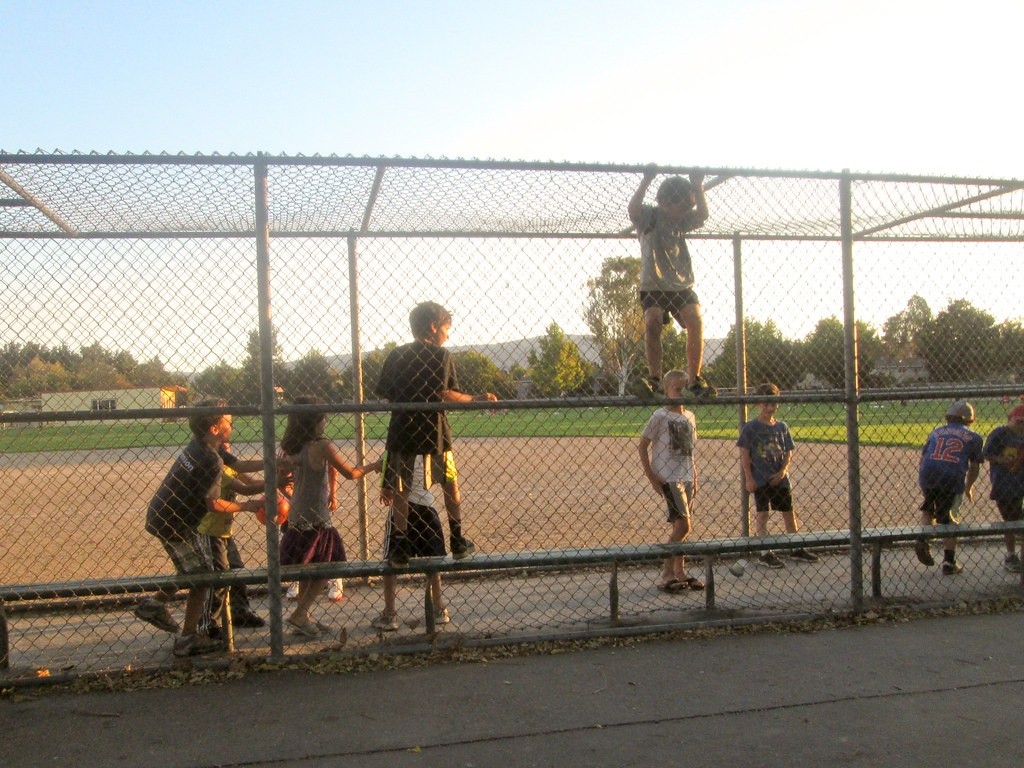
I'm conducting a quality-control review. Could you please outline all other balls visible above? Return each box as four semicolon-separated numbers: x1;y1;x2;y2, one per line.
257;494;289;526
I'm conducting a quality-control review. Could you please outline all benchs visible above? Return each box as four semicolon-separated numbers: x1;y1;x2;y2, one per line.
0;519;1024;681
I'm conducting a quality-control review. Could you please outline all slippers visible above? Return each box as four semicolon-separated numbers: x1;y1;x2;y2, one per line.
658;578;690;592
684;577;705;590
292;621;332;635
284;617;322;638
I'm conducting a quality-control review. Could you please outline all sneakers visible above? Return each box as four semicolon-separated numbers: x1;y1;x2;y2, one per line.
134;599;180;632
286;579;300;600
327;578;343;601
173;633;224;655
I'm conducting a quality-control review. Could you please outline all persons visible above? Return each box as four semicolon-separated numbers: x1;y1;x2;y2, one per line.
736;384;819;567
278;396;384;638
983;404;1024;571
638;370;705;591
372;448;450;629
916;402;984;573
627;162;718;399
133;398;295;655
196;444;266;637
377;303;497;568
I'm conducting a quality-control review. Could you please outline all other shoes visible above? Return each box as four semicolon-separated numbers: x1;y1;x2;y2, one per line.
434;607;450;624
760;553;784;569
914;540;935;566
682;377;717;399
632;377;663;399
231;608;267;628
199;616;224;639
1004;555;1024;572
942;560;963;574
790;548;819;563
372;615;398;629
449;535;476;559
387;544;411;570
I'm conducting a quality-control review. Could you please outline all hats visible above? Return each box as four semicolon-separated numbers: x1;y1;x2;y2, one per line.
1010;404;1024;423
946;401;975;422
